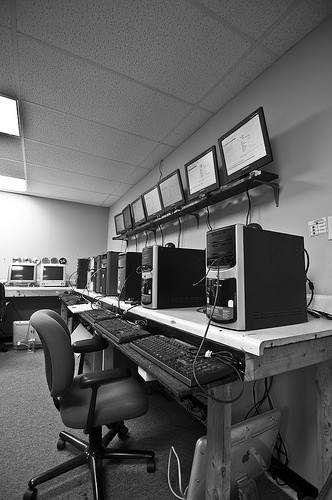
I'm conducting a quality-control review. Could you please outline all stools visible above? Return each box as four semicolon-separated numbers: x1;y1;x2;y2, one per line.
70;330;109;375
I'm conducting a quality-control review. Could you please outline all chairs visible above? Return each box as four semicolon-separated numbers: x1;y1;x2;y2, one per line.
23;308;155;500
0;282;17;353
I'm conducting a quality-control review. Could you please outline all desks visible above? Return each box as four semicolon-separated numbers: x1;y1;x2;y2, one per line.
0;287;332;500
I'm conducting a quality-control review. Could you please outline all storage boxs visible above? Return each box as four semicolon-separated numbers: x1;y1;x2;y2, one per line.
14;321;41;344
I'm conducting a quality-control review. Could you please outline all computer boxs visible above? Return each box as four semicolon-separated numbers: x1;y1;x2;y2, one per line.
204;223;307;330
100;252;116;296
117;251;139;301
93;254;107;293
76;259;93;288
140;244;205;310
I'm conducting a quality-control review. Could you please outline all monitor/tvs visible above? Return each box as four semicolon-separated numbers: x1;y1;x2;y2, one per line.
184;145;219;200
8;264;36;287
13;320;41;353
121;204;132;230
157;168;186;212
141;184;165;221
129;195;146;227
184;408;280;500
113;212;127;235
39;264;66;286
218;107;273;184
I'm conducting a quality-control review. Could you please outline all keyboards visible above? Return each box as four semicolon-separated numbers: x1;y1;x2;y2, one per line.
59;292;91;304
83;308;121;323
95;318;150;344
133;334;239;386
